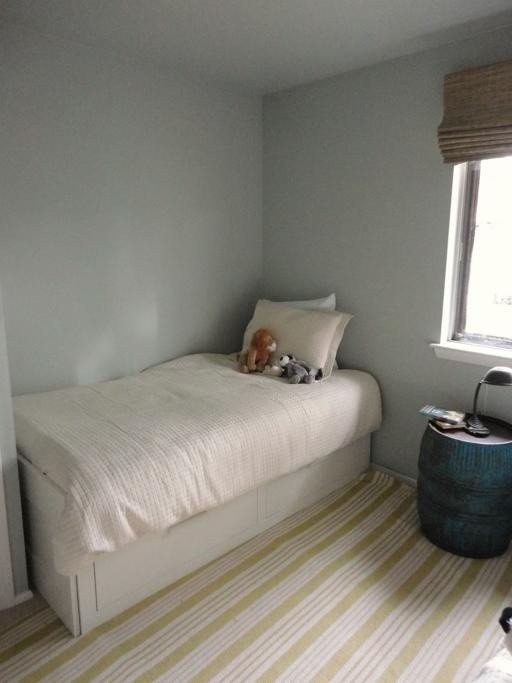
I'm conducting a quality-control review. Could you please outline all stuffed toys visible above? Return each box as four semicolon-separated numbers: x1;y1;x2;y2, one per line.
239;329;277;374
279;354;322;384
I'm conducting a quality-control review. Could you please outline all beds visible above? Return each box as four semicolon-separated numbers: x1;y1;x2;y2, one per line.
8;350;385;639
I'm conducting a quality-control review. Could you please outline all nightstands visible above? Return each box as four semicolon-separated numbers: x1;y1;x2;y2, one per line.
412;408;512;560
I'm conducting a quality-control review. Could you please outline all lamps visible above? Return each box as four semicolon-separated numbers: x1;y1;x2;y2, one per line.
462;362;508;437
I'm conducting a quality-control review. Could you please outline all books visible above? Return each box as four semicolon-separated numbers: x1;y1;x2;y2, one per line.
419;404;466;424
427;416;467;430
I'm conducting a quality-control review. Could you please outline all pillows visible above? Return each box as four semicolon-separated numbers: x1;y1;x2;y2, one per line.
241;299;351;382
276;292;347;312
